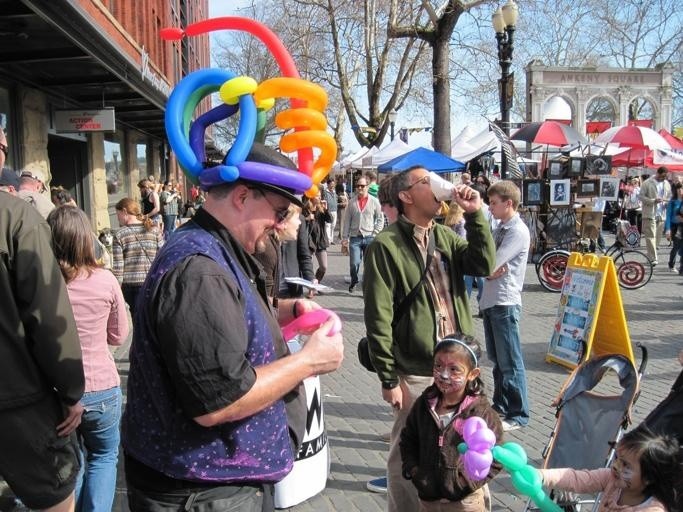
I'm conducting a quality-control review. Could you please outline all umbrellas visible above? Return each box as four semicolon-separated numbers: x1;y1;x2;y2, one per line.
508;119;683;188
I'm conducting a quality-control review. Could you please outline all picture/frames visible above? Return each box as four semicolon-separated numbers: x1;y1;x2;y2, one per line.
568;157;584;176
577;179;600;198
550;179;571;206
600;178;621;202
523;180;545;206
549;160;563;180
586;155;611;174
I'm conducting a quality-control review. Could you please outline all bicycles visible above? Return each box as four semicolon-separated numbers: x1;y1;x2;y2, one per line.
535;225;653;292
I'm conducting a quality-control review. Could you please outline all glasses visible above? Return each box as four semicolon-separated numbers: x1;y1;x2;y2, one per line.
409;176;430;188
260;189;288;222
0;143;9;156
356;184;367;188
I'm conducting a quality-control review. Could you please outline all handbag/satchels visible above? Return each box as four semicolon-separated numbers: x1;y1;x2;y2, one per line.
358;338;377;372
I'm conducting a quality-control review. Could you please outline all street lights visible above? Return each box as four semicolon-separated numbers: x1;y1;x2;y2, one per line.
492;0;518;180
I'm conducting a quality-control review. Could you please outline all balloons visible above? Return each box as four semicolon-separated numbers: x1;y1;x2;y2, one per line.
158;17;339;200
456;416;563;511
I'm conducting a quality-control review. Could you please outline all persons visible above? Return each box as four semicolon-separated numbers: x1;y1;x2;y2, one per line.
1;142;346;511
602;166;683;274
337;164;529;511
532;420;683;512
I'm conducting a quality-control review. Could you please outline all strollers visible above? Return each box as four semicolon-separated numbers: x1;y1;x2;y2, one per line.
523;333;646;512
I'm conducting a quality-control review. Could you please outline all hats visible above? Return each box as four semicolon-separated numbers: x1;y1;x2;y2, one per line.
0;168;48;191
222;142;304;206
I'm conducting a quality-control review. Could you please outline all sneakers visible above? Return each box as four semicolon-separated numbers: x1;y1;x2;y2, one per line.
367;478;388;493
502;419;521;432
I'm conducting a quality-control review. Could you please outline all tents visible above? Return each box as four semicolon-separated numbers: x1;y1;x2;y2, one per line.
332;141;413;198
377;147;465;185
